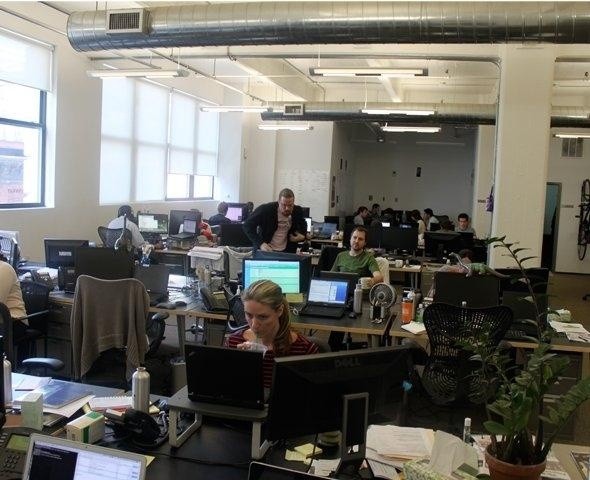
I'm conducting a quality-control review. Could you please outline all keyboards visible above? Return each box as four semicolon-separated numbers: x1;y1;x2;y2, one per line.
504;330;526;339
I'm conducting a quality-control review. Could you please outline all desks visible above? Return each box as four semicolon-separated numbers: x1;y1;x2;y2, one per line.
1;259;590;443
1;366;587;480
88;237;494;299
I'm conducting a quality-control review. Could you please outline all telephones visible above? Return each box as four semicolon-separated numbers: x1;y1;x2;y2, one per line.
30;269;54;290
0;427;53;480
200;287;230;311
58;266;77;294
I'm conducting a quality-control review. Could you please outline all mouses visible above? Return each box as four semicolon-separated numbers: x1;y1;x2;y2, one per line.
348;311;357;319
544;335;550;343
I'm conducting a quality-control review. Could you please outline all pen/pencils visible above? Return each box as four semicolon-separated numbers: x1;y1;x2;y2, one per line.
15;389;34;391
149;399;160;408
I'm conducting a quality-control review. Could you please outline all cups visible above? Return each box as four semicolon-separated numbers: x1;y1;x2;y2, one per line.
358;277;371;289
395;259;403;268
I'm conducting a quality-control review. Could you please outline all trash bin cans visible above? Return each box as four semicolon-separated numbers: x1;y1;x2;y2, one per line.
169;357;188;397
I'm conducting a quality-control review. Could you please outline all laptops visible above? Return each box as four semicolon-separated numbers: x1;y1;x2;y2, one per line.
184;343;268;410
300;275;351;321
21;432;148;480
134;265;169;305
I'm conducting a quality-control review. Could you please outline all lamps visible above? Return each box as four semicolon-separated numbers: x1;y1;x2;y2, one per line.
201;44;442;137
86;48;190;79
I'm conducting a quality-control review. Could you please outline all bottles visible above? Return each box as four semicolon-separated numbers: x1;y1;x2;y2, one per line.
407;288;416;321
353;284;364;315
4;355;13;405
131;366;151;413
415;303;425;321
204;264;211;286
401;297;412;324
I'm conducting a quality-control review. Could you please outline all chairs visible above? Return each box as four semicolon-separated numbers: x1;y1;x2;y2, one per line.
95;205;483;269
493;266;552;325
429;275;502;324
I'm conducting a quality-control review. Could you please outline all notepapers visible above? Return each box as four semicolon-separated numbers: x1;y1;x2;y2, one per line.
294;443;323;458
149;401;160;414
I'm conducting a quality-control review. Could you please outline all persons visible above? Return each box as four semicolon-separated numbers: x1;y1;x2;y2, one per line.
208;202;231;225
353;204;474;246
242;189;307;256
191;209;212;240
328;226;383;352
105;205;164;255
0;245;29;345
223;280;320;403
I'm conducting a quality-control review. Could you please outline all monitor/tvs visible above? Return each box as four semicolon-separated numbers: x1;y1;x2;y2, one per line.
9;238;18;270
248;460;336;480
129;195;473;260
44;240;87;267
242;259;299;293
503;291;547;325
262;345;413;478
434;271;498;308
